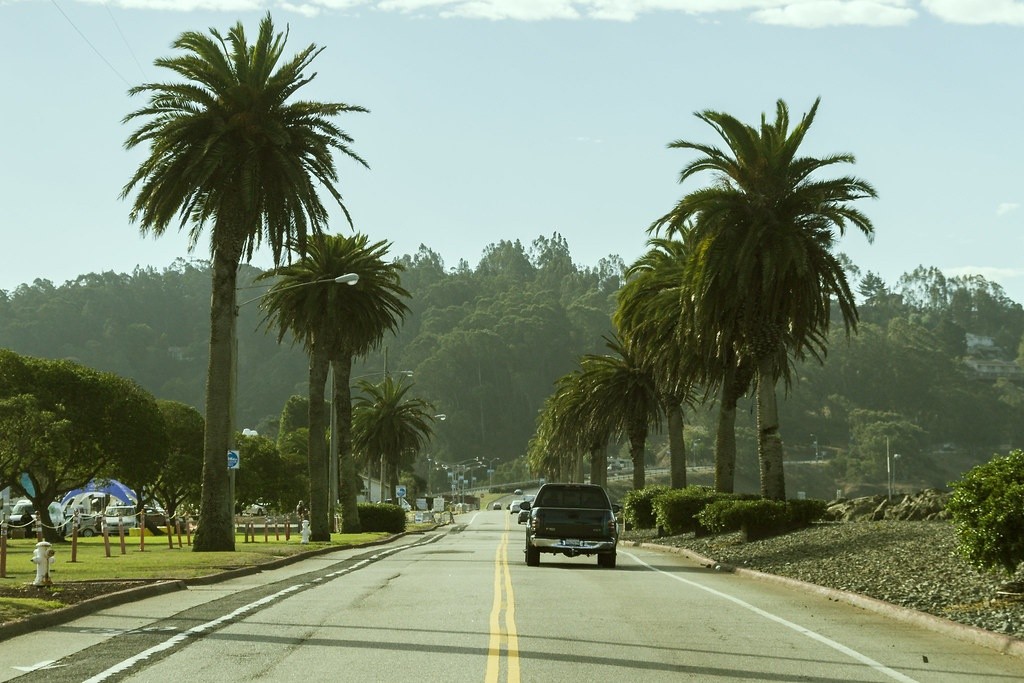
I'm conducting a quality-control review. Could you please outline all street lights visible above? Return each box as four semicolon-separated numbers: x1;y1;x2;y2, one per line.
482;456;500;491
233;272;360;537
426;454;485;505
810;433;820;462
328;363;414;533
693;439;701;467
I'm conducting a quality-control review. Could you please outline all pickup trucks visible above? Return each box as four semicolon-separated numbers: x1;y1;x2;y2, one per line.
520;482;621;568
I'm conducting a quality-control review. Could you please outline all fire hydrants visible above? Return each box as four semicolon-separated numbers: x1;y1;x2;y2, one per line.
299;519;313;544
30;539;58;587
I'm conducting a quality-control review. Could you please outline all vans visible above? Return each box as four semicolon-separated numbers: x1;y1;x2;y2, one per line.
99;505;137;536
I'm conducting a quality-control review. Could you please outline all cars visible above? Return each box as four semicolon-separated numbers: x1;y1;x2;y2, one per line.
377;498;412;513
522;496;536;508
142;506;199;534
510;500;523;514
515;489;523;495
517;508;531;525
493;503;502;510
243;504;267;517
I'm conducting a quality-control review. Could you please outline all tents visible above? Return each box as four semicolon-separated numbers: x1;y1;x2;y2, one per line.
61;478;139;512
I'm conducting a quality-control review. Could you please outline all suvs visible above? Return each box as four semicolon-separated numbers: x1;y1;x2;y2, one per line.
7;501;68;538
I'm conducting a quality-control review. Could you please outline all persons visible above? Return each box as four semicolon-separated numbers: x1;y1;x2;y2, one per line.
35;511;42;531
296;500;304;533
75;508;81;529
21;511;35;539
